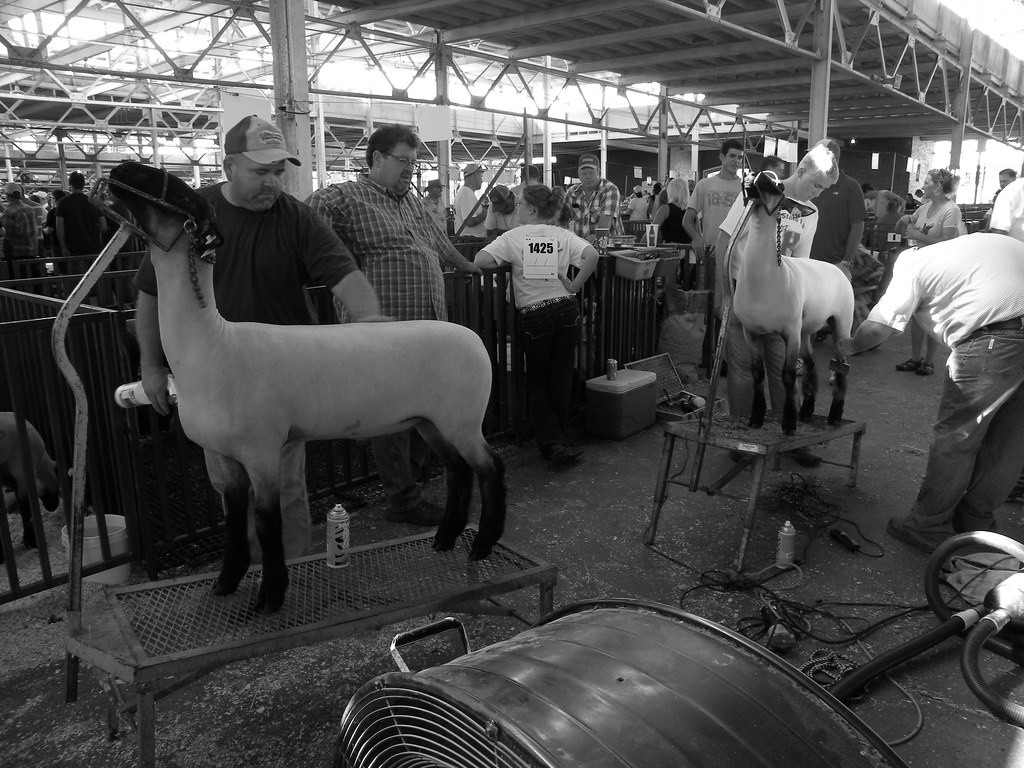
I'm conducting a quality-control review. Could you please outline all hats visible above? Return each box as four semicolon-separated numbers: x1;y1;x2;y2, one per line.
4;183;22;197
579;154;599;170
423;179;446;192
32;191;47;198
490;186;512;212
634;186;643;194
224;115;302;167
464;164;486;176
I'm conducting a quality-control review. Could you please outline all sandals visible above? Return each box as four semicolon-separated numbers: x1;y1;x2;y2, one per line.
896;358;920;370
918;362;933;374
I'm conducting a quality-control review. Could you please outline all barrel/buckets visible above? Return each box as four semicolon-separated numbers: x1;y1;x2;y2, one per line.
62;514;132;585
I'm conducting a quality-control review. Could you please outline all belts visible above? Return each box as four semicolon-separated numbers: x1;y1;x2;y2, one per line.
520;296;574;315
977;316;1023;329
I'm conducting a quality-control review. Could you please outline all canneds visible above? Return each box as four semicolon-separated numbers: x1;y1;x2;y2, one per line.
607;359;616;380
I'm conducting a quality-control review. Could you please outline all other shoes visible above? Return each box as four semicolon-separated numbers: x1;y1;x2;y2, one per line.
791;446;822;465
887;517;934;552
552;445;583;464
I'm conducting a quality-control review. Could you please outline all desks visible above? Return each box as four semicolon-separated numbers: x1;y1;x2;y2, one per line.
100;524;561;768
643;410;866;592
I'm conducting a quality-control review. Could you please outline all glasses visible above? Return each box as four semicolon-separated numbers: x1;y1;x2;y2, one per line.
516;202;536;213
380;151;419;169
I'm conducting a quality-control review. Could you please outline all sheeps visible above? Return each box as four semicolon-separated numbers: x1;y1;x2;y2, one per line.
731;172;855;438
107;159;507;615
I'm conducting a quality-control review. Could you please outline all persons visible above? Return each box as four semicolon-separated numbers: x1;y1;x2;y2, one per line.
130;116;391;558
476;184;598;464
415;138;1024;290
0;172;140;311
306;126;482;525
841;231;1024;550
716;147;841;466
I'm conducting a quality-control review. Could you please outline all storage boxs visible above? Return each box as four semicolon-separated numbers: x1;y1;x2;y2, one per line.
653;255;681;278
583;367;658;442
624;352;726;425
608;250;659;281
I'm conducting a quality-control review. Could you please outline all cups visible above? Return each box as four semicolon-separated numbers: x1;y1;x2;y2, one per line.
594;227;608;255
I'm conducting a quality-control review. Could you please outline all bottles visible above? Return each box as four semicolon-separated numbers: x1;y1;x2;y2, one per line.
446;208;456;239
775;520;796;568
680;389;705;407
907;223;917;246
326;504;351;568
114;376;178;409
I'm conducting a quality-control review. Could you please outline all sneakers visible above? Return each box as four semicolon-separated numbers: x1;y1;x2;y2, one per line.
384;501;445;525
